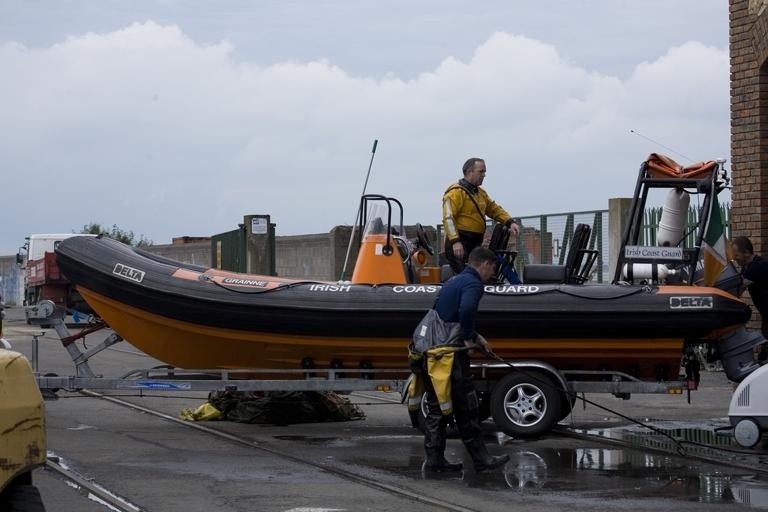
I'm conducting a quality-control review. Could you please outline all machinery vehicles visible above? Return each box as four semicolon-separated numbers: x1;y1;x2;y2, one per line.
0;348;51;512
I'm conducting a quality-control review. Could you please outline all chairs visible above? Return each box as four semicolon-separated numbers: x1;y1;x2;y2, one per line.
440;224;510;281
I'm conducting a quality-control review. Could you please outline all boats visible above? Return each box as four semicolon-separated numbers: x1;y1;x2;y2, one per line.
51;129;766;385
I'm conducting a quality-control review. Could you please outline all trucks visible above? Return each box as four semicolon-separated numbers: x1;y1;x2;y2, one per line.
15;231;102;330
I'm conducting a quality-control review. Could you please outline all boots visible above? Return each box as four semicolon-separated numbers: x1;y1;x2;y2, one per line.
421;413;463;474
457;421;511;474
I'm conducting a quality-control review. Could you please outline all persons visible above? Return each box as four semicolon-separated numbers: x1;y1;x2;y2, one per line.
730;235;768;366
413;243;513;473
439;157;521;275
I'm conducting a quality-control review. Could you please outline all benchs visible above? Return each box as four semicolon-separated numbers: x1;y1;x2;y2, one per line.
523;223;590;283
